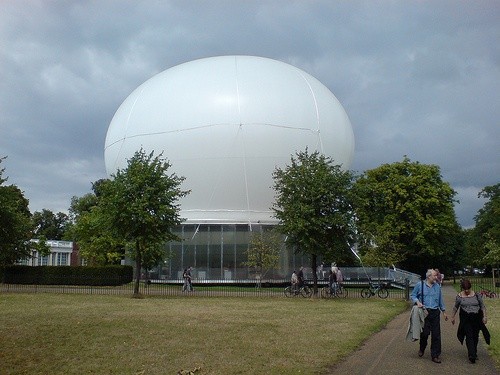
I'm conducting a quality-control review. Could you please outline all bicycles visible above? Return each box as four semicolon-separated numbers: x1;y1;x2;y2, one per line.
361;277;388;299
475;286;496;298
284;280;313;298
321;281;348;298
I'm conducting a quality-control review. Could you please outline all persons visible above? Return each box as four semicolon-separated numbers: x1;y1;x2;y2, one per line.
451;279;491;364
327;263;343;297
495;280;500;295
142;270;151;289
410;269;449;363
290;264;311;295
181;267;194;292
435;269;442;285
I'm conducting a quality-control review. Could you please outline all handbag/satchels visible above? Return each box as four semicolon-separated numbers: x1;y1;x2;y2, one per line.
475;292;484;319
422;306;428;318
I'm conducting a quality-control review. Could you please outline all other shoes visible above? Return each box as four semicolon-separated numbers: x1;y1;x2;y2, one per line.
418;350;424;357
468;353;479;363
432;356;441;364
